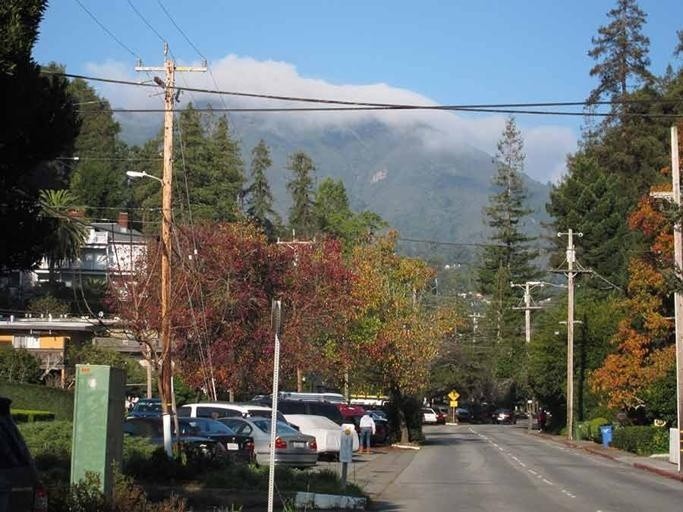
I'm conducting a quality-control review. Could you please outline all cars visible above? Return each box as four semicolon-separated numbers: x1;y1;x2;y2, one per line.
250;392;395;446
282;413;359;461
214;416;317;468
0;397;62;511
421;407;437;424
432;408;447;425
132;398;162;417
455;402;519;424
121;417;254;468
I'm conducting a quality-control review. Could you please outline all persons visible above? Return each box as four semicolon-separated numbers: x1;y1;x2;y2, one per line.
533;409;547;433
359;414;376;453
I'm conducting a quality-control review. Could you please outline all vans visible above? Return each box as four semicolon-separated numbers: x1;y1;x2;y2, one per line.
170;401;289;428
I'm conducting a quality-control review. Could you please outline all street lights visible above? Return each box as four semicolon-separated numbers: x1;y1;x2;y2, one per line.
125;171;172;412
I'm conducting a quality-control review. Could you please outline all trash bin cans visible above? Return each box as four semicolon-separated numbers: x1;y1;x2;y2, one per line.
600;426;613;447
